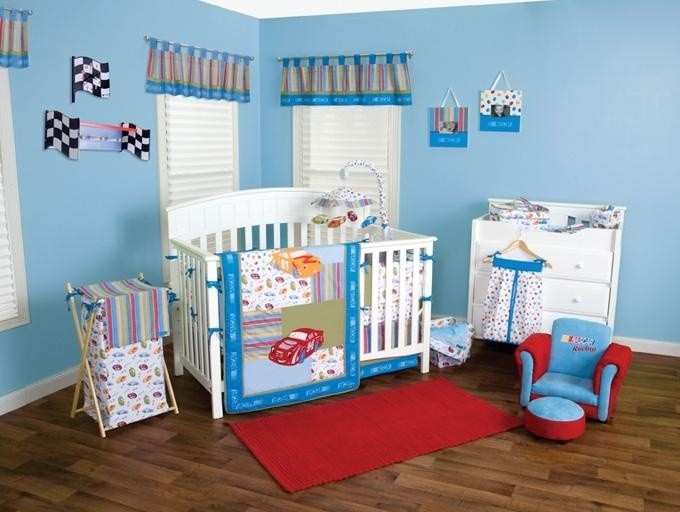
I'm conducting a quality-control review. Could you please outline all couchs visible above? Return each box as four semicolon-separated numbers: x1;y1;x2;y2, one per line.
512;316;633;423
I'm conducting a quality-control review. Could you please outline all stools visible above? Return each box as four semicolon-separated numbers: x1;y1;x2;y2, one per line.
522;395;587;446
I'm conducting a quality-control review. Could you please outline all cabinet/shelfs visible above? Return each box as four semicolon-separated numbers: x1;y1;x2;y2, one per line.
464;198;628;349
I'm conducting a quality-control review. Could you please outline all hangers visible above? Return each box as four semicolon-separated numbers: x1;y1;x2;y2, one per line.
481;229;553;271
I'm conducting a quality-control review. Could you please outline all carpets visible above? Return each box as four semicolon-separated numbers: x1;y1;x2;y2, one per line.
222;371;524;496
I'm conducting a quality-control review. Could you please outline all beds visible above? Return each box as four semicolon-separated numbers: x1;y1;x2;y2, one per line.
163;186;437;420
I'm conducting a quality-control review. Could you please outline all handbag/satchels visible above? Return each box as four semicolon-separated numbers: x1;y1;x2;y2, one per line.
490;196;549;231
